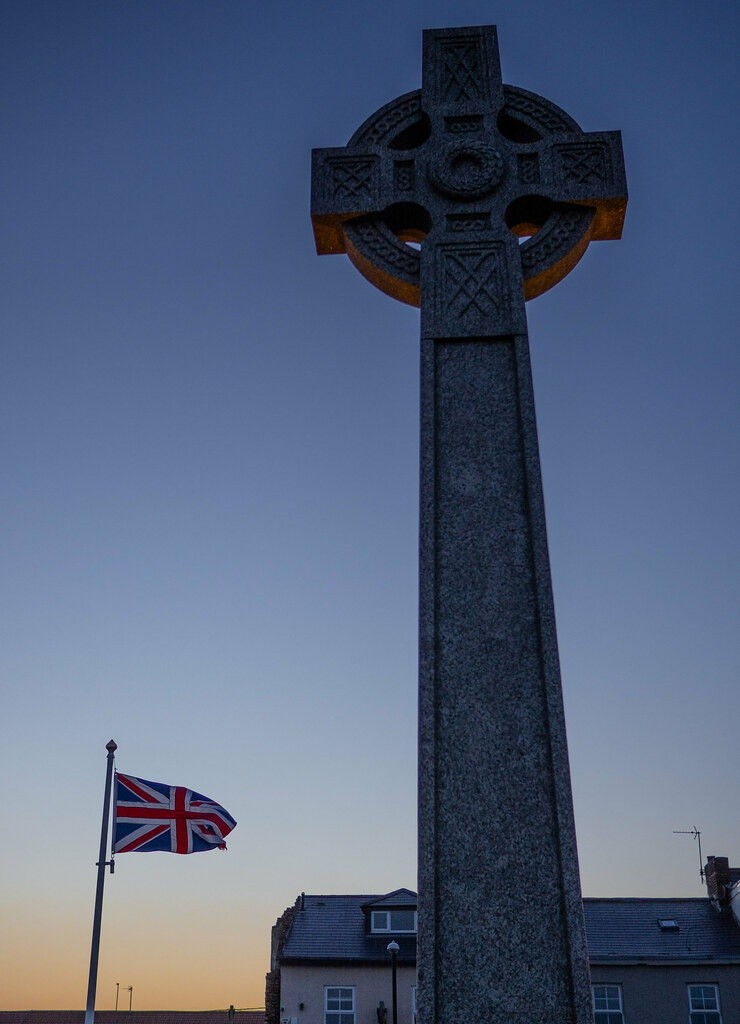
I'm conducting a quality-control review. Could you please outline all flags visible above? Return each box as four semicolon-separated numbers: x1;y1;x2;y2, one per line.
113;772;237;854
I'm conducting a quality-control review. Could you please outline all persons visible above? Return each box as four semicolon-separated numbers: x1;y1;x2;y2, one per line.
228;1006;235;1024
377;1001;387;1024
